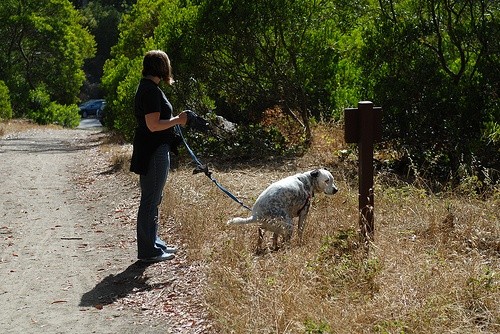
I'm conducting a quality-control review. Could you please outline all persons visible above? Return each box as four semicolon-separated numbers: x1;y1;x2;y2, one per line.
129;49;187;263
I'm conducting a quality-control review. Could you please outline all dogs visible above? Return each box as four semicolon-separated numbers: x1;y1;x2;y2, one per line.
226;166;338;250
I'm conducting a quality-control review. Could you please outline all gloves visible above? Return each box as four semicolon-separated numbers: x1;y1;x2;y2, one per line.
183;110;209;133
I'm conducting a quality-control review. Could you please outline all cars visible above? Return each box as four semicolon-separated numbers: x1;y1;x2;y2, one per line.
78;99;106;118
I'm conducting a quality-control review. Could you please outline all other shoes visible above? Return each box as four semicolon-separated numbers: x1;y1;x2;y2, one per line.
142;252;175;262
163;247;177;253
